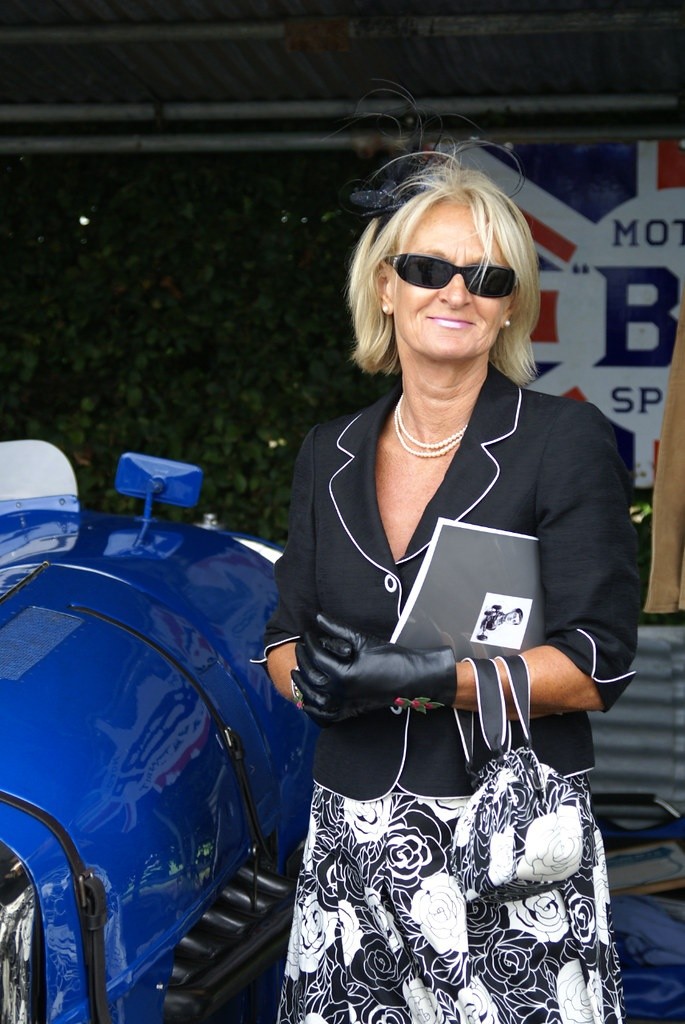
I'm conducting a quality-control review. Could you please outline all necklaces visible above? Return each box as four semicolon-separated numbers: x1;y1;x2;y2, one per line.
393;394;467;457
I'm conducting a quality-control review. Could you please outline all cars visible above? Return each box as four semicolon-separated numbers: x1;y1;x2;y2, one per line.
1;439;322;1024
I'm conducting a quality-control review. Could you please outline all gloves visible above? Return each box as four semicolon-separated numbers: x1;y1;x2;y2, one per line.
290;613;457;727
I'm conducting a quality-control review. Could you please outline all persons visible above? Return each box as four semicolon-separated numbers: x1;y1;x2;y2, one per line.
248;145;644;1024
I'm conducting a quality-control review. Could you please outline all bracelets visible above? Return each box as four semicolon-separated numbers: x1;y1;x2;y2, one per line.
290;666;301;699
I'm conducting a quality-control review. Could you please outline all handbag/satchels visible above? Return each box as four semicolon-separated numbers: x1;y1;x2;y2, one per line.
452;654;584;902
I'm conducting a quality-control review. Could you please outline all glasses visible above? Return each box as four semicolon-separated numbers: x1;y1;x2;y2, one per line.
384;253;518;298
